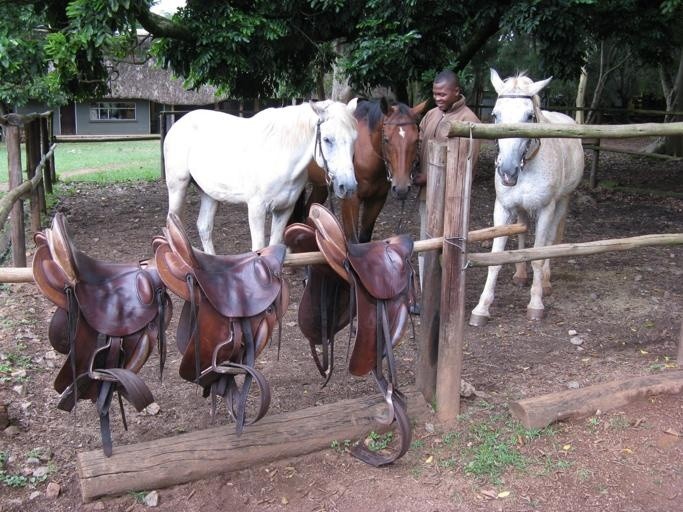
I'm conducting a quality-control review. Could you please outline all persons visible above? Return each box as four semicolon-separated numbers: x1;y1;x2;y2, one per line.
408;71;485;318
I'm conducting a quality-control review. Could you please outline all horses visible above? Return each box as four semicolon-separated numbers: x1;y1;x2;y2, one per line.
158;95;363;256
470;66;586;327
286;83;428;245
